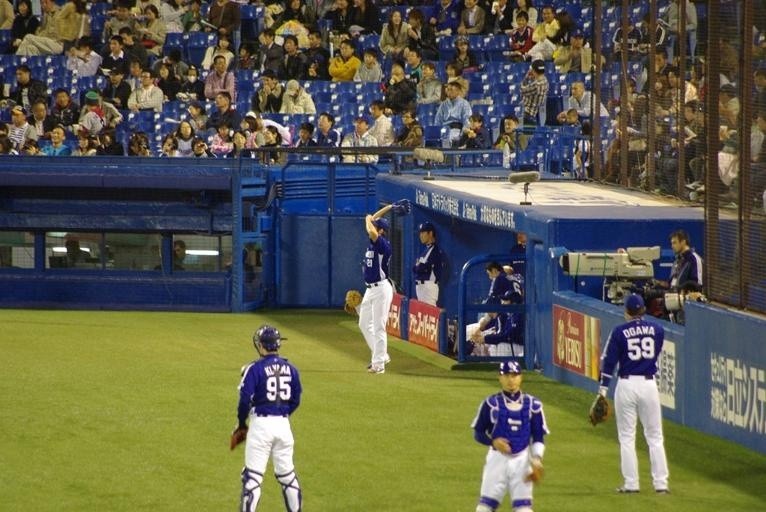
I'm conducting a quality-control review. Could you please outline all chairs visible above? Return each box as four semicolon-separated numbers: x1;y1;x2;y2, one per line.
0;1;765;168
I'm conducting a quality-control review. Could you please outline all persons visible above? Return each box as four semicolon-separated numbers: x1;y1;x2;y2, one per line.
311;113;342;147
555;110;581;155
452;37;482;72
542;12;587;46
306;30;329;81
398;111;427;166
164;92;286;165
329;40;359;84
59;238;92;271
4;105;38;149
152;241;198;276
340;117;379;164
96;126;124;156
177;67;204;101
71;131;96;158
128;71;163;111
403;49;423;77
202;31;232;71
151;48;189;79
415;64;444;105
41;124;72;156
652;231;704;327
224;247;257;303
126;57;145;86
161;0;190;32
358;204;394;373
517;60;548;129
135;0;161;18
235;43;256;72
256;31;283;78
101;36;127;70
495;116;529;168
263;0;379;41
429;0;457;37
49;88;83;125
278;79;316;115
457;264;526;359
67;36;103;79
205;56;233;100
414;222;441;305
511;0;539;28
10;0;39;52
520;5;559;62
557;83;612;118
157;64;180;99
100;68;127;109
408;9;439;60
509;11;535;62
433;80;470;151
487;0;510;33
0;0;15;30
19;139;49;156
0;135;18;155
9;67;49;107
553;29;606;73
253;68;282;116
135;5;167;57
79;88;123;127
182;0;206;31
119;26;147;66
355;48;381;86
444;61;469;99
367;100;396;148
457;115;492;151
59;0;91;50
25;100;59;139
379;11;411;61
102;0;138;48
597;294;671;494
609;1;766;208
470;361;549;512
457;0;485;36
282;35;307;79
203;0;242;34
382;61;415;114
509;232;527;264
17;0;65;56
294;123;316;159
127;133;153;156
69;91;107;135
233;325;301;511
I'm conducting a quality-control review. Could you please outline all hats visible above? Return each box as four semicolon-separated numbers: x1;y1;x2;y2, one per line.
11;105;27;115
572;29;584;37
531;60;545;70
371;218;389;230
498;291;520;301
253;326;280;348
108;67;124;74
418;222;433;232
286;80;300;96
498;362;521;375
679;281;702;292
624;294;644;310
455;36;470;44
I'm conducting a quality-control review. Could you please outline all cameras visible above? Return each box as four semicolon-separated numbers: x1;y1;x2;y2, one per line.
128;133;142;146
87;134;104;141
558;245;659;277
664;292;706;311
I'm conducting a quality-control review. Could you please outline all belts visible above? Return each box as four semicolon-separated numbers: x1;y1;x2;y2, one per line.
257;413;289;418
365;279;388;288
619;374;655;381
415;280;438;285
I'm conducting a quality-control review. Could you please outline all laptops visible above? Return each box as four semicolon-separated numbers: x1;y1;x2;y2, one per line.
49;256;68;269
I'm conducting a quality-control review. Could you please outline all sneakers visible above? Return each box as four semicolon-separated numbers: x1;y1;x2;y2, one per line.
616;486;640;493
368;357;392;368
368;366;383;374
655;487;670;494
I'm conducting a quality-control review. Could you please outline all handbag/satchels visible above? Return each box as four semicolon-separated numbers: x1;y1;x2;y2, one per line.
139;20;157;49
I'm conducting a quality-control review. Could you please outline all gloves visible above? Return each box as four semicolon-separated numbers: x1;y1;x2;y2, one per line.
590;396;610;424
523;457;544;481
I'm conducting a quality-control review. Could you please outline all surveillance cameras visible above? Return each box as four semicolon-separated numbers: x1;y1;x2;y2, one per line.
508;171;540;185
414;148;444;163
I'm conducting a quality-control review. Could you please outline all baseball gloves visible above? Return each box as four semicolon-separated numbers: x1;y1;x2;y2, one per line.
589;395;610;427
522;459;543;483
392;199;411;215
